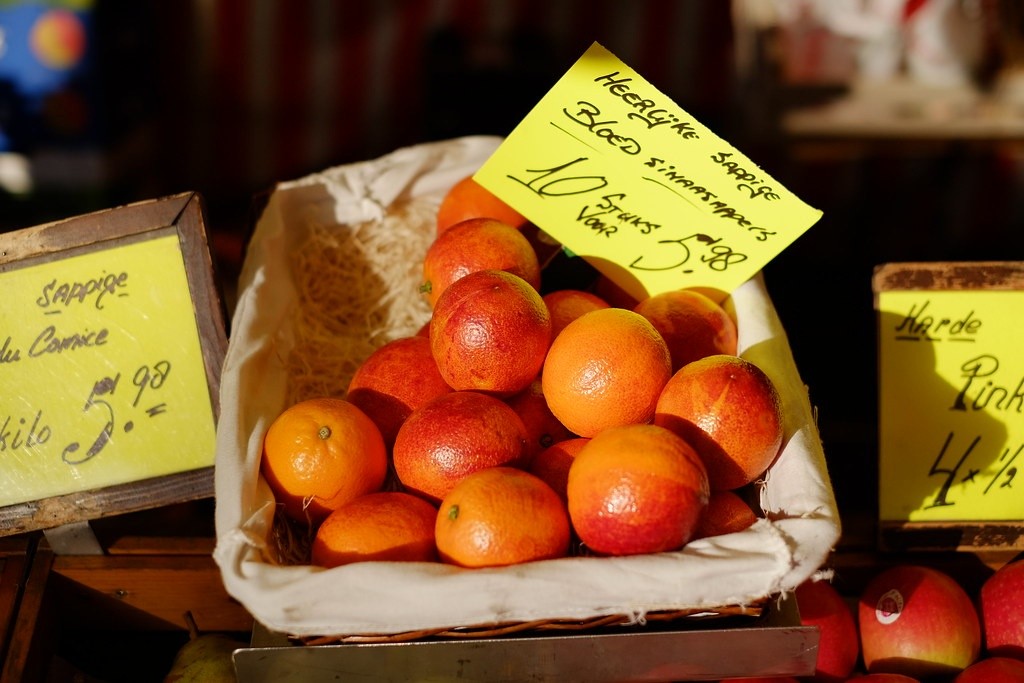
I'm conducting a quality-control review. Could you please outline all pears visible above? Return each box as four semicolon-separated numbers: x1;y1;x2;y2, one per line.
164;609;248;683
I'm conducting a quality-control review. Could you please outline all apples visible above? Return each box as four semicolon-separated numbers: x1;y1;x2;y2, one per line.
720;557;1024;682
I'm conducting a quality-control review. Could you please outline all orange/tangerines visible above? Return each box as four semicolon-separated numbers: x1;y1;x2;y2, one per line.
256;174;780;566
